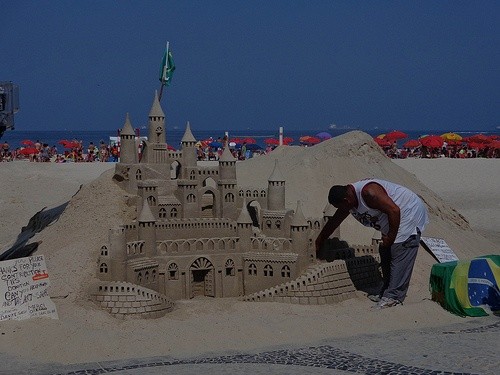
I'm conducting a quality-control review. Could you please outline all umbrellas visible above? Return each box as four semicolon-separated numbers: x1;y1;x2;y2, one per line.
19;146;38;154
195;136;262;149
64;142;79;148
60;139;70;144
22;139;35;146
373;130;500;149
265;132;331;146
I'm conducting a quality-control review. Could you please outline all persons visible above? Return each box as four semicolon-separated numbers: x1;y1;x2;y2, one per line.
198;141;314;161
381;140;500;159
0;137;142;163
315;178;429;308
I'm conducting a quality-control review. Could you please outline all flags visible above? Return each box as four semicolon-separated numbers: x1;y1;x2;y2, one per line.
159;48;176;87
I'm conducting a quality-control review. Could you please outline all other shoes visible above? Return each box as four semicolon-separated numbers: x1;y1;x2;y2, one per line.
377;297;400;307
367;294;381;302
